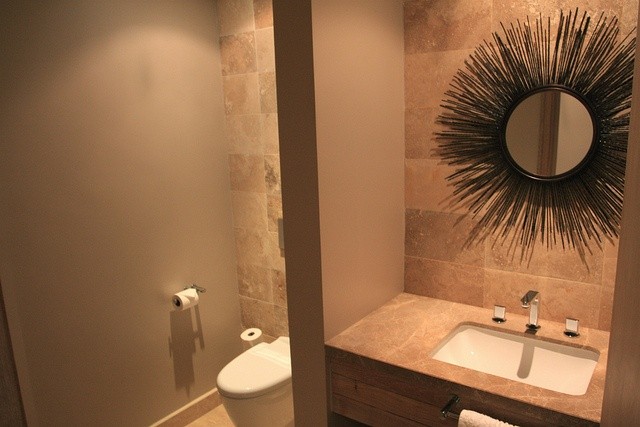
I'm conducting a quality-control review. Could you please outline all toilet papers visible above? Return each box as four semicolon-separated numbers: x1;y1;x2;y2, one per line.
171;287;201;311
238;327;264;346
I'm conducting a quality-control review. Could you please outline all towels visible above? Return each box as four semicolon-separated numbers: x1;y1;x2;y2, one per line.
456;409;519;426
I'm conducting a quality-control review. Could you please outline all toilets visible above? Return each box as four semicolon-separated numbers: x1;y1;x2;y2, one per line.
216;336;293;426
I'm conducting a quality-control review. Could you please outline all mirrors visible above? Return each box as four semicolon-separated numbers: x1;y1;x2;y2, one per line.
499;84;601;186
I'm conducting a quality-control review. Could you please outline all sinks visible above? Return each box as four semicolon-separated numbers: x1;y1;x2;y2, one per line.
427;320;603;396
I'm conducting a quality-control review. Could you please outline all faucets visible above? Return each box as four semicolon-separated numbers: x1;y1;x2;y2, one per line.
520;289;540;334
